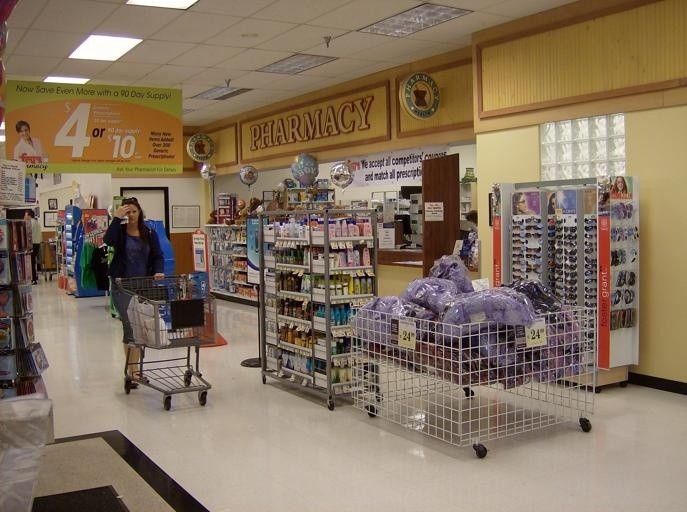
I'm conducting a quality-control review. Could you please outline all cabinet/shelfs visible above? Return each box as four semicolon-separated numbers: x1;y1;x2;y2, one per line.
202;222;262;302
253;209;378;417
506;186;629;393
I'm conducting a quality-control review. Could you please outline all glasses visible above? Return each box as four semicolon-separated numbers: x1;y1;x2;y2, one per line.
122;197;138;204
509;213;596;357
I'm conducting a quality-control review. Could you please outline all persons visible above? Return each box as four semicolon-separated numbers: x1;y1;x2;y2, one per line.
236;200;245;217
466;210;478;243
548;193;556;214
250;198;264;213
24;210;42;285
599;192;610;210
512;192;535;215
103;197;164;383
207;210;219;224
14;121;42;161
611;176;628;194
288;205;347;218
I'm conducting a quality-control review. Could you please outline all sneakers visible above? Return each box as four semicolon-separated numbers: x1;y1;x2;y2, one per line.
30;279;37;286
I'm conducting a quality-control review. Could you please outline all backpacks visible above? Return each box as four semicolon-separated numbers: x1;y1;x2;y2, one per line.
266;193;279;221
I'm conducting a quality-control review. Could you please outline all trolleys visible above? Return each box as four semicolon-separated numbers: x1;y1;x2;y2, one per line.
112;274;217;410
